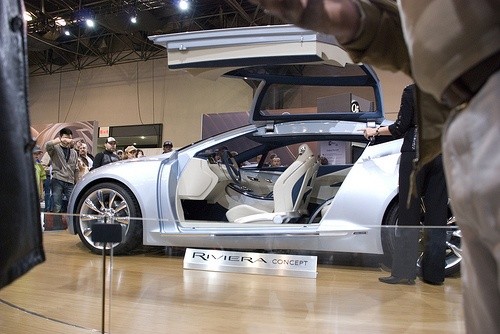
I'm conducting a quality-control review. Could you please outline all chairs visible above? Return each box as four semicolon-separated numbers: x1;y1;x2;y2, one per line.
225;143;321;224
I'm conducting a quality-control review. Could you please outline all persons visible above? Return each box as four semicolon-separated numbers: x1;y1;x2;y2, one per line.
207;148;283;169
362;82;449;287
251;1;500;334
31;127;175;213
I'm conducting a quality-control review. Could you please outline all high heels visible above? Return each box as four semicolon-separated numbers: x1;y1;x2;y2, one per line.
378;272;414;285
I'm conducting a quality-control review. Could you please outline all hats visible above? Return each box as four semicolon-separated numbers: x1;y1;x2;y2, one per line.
105;137;116;142
125;146;137;152
32;145;44;154
163;140;173;148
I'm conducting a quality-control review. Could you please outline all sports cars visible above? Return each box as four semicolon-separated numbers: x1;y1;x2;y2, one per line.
67;23;463;278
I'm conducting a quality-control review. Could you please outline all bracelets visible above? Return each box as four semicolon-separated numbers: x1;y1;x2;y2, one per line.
374;126;380;136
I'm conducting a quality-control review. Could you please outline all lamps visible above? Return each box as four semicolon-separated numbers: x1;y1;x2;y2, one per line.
84;8;97;28
64;26;72;36
130;6;141;24
